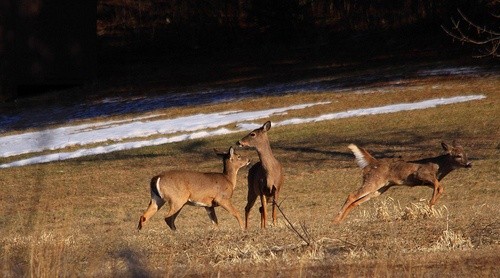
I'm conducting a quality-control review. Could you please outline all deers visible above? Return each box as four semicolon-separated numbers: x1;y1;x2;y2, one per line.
330;139;473;225
235;120;282;229
137;146;251;233
